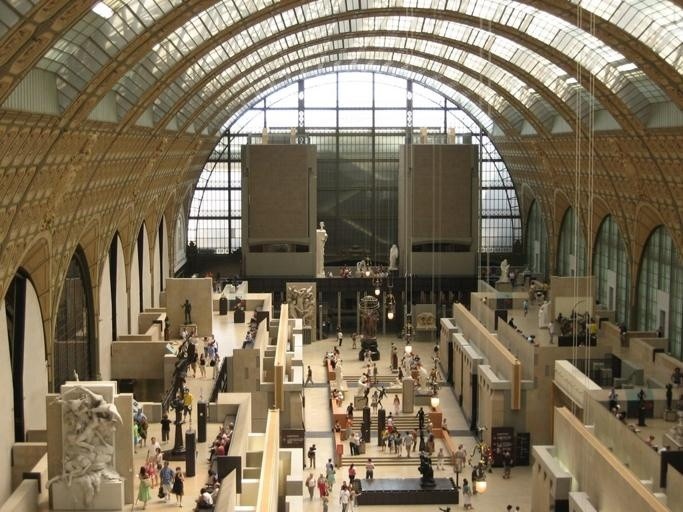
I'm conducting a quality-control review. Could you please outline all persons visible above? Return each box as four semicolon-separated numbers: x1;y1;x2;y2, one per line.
609;367;683;455
305;320;439;415
132;328;235;511
332;403;425;480
482;273;597;347
180;300;192;325
500;259;510;282
243;309;258;347
427;417;513;496
305;446;356;511
389;244;399;269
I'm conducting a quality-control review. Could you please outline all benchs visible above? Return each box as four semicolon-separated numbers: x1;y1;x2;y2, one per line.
241;302;263;349
193;413;235;512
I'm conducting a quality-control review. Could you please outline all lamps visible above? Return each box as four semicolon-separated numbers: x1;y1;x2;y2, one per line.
365;3;594;512
